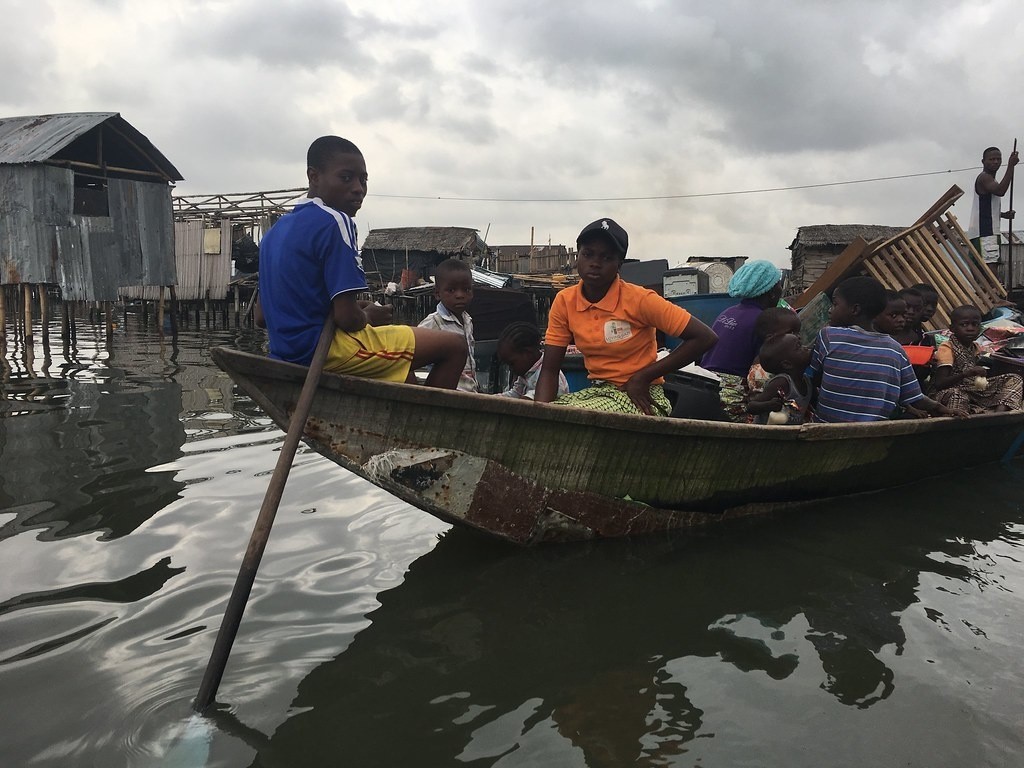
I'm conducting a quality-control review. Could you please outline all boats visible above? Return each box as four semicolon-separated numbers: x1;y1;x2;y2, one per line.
204;338;1024;546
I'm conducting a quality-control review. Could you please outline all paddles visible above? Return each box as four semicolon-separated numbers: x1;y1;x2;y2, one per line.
191;310;337;713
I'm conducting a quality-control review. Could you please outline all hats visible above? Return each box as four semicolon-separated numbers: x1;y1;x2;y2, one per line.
577;218;628;259
729;261;780;299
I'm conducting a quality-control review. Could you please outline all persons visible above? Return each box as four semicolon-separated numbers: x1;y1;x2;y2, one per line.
416;259;483;393
493;321;570;402
701;260;1024;419
968;147;1019;287
533;218;720;417
258;136;469;390
